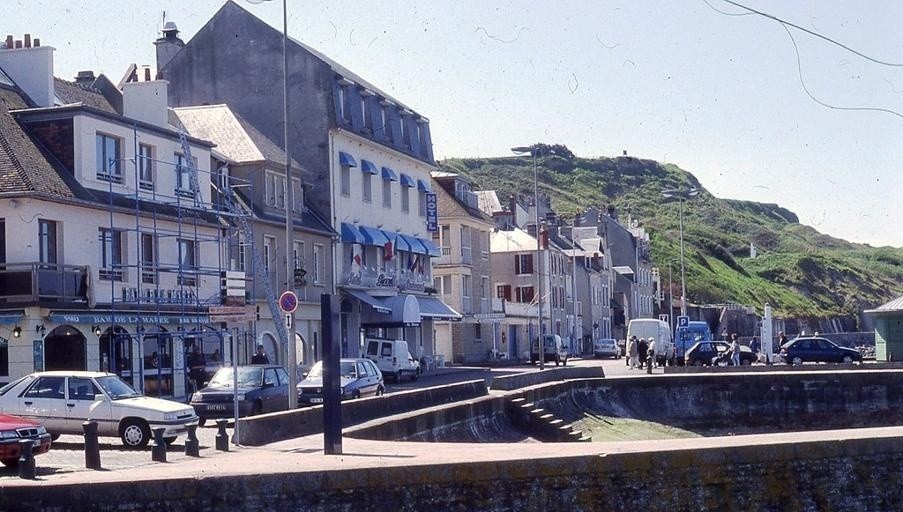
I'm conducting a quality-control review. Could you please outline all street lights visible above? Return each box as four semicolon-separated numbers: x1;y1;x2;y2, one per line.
510;144;547;372
661;185;700;334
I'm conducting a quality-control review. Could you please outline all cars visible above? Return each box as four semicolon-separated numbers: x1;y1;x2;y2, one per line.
188;362;300;428
777;336;863;367
592;337;624;360
673;339;758;366
0;409;53;471
294;355;386;410
0;367;201;450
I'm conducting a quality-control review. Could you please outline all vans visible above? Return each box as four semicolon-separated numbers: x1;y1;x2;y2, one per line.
672;319;714;366
528;332;568;368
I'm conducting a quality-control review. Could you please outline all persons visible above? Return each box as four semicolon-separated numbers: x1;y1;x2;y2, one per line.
627;334;658;370
776;329;820;354
151;345;220;392
711;327;740;374
749;335;759;363
252;345;270;365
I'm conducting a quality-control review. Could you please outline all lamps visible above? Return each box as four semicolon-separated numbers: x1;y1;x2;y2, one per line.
13;324;21;338
92;325;101;336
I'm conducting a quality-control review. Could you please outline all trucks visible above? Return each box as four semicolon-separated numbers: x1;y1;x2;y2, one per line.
361;334;422;383
624;318;676;367
